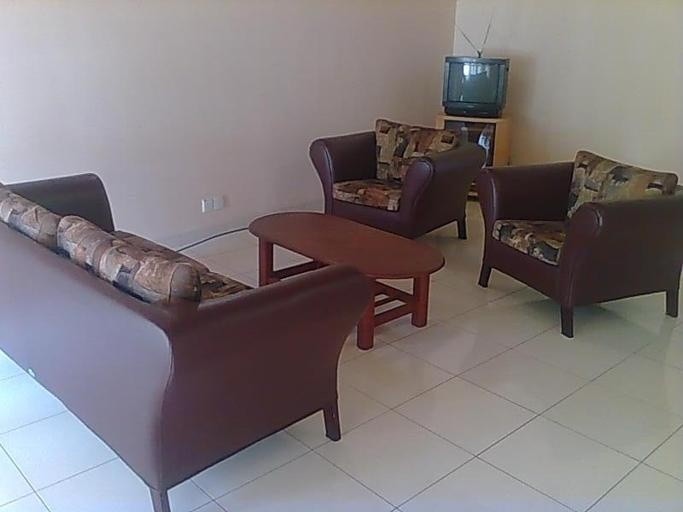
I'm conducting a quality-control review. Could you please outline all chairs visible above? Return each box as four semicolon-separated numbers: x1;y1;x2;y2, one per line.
474;160;681;338
307;131;486;240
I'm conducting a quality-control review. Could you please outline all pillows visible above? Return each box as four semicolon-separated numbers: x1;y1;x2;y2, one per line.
566;149;678;224
54;214;201;307
0;181;60;256
373;118;456;187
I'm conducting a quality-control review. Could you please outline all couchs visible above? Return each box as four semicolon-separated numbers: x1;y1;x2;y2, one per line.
0;171;380;512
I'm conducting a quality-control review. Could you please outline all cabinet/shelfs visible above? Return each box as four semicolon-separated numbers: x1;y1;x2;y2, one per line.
434;115;512;198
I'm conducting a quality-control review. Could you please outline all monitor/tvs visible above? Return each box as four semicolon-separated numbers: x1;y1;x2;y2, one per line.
442;57;510;118
